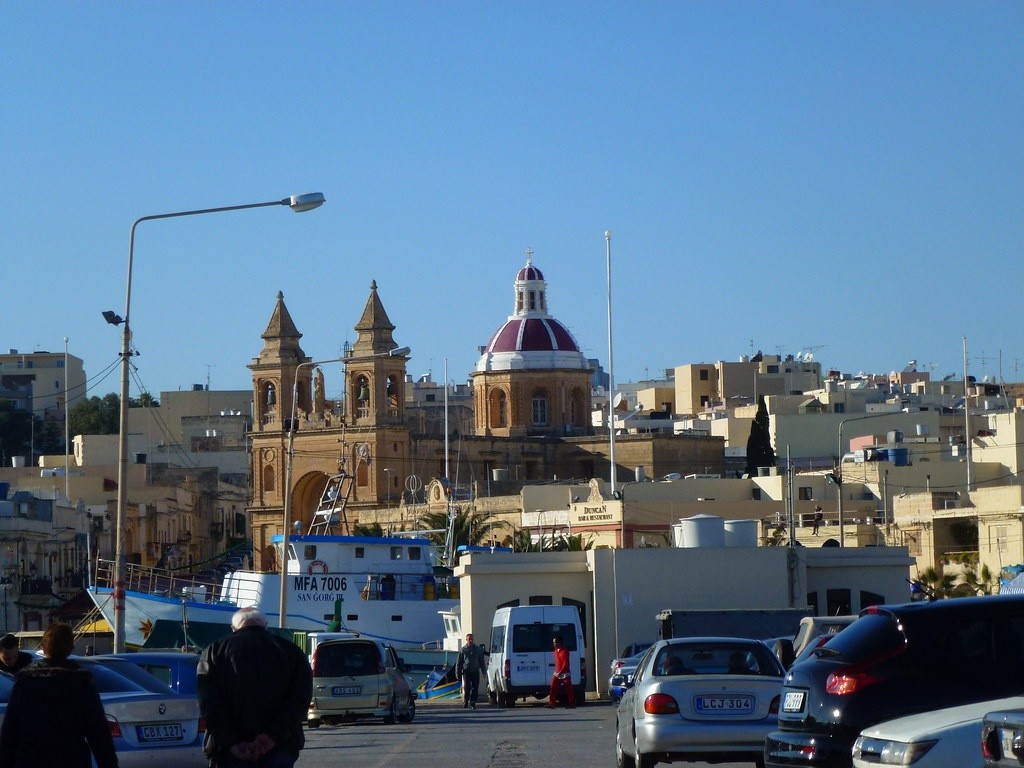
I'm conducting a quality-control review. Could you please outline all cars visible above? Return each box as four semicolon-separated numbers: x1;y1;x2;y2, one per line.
307;638;416;728
606;642;667;700
764;592;1024;768
0;645;208;768
856;695;1024;768
610;637;786;768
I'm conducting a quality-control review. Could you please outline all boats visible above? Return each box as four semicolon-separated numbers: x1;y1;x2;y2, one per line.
84;360;511;652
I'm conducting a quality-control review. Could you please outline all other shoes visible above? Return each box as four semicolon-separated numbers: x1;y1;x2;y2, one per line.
463;705;467;708
472;706;476;709
565;705;574;709
544;705;555;709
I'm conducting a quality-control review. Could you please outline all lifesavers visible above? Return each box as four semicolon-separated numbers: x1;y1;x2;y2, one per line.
308;561;329;574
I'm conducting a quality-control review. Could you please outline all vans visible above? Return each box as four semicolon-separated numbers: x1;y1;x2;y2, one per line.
478;604;589;708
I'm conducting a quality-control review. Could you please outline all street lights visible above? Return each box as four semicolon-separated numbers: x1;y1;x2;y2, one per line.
605;230;620;494
279;343;411;629
826;406;923;549
63;336;70;500
100;191;327;654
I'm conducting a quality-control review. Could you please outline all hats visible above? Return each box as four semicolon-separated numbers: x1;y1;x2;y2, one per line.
0;632;18;649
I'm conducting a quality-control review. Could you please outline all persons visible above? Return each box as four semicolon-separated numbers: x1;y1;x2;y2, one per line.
195;607;313;768
0;634;35;714
455;634;487;709
544;635;576;709
0;622;119;768
814;505;823;520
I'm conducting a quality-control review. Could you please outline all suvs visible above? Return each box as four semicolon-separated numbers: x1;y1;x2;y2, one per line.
772;615;858;669
